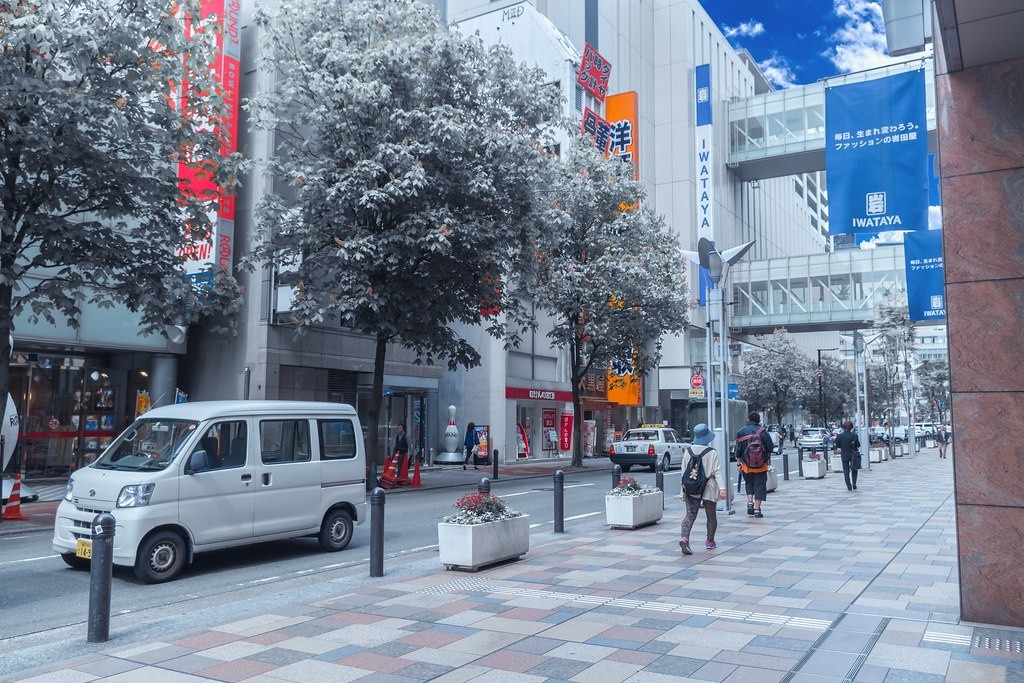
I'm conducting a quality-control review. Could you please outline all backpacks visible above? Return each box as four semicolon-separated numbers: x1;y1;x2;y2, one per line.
736;428;766;468
681;446;715;495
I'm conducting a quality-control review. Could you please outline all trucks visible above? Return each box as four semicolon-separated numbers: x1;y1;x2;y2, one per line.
684;395;749;463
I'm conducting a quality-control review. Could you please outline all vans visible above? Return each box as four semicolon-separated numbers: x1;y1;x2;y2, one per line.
51;400;367;585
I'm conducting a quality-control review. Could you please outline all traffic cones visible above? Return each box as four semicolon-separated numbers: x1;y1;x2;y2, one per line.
1;473;30;520
379;451;400;489
396;453;411;485
407;457;424;488
384;456;390;473
366;461;380;490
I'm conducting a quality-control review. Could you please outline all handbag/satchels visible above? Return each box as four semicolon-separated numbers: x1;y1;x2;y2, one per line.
471;445;480;456
851;450;861;471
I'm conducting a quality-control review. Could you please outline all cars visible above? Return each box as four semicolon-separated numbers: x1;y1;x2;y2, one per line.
610;424;691;473
797;427;834;451
832;423;952;449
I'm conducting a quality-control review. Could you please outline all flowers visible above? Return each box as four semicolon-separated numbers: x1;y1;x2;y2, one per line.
801;451;825;463
441;488;527;525
606;475;661;497
870;440;900;449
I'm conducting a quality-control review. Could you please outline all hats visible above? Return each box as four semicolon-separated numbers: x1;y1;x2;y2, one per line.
691;423;715;444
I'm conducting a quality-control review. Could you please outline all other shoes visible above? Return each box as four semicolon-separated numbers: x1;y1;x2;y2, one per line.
754;509;763;517
463;465;467;471
474;466;480;470
747;501;754;515
852;484;858;490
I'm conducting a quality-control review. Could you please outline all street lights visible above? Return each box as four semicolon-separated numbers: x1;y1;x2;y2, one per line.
674;236;757;516
817;347;840;428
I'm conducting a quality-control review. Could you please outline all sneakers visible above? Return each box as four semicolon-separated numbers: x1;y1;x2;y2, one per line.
706;541;717;549
679;537;692;554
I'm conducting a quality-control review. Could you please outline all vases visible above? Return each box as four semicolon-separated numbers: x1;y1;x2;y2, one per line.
830;458;850;472
869;442;920;462
765;469;777;493
802;460;827;479
605;491;663;530
438;514;531;571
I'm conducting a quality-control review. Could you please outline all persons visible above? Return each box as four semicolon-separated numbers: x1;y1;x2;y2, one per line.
935;425;950;458
789;423;796;442
462;422;482;470
679;422;727;555
835;421;863;491
734;413;774;519
391;423;413;478
781;424;787;435
